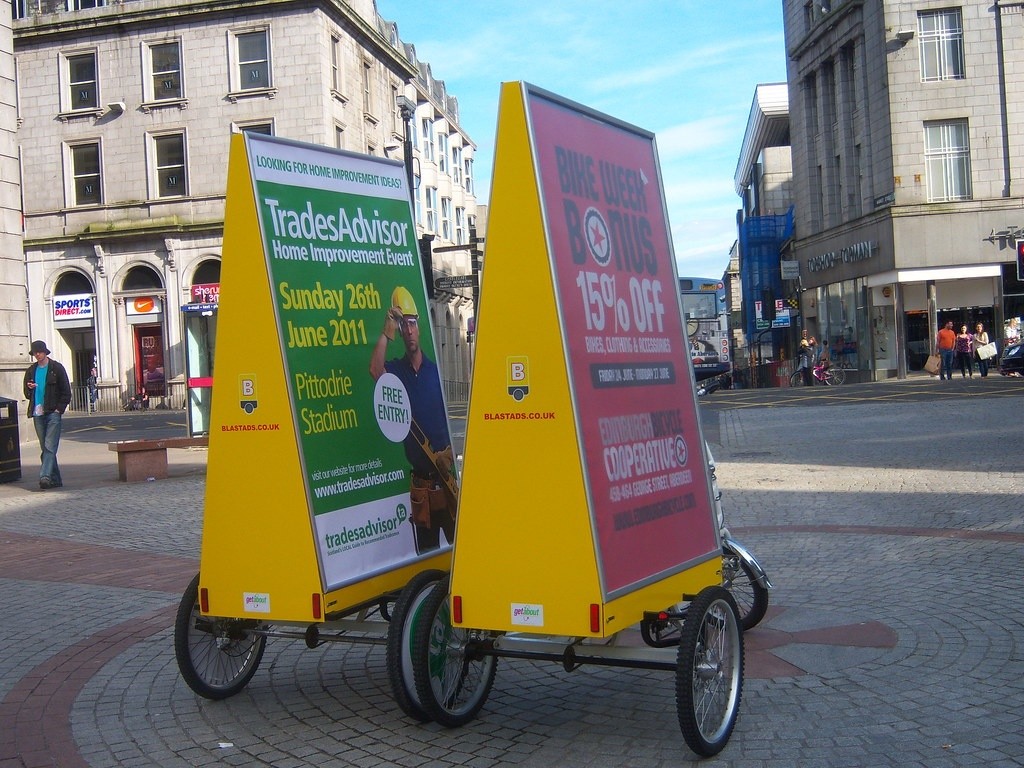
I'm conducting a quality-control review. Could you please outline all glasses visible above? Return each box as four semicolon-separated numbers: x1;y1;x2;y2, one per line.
399;319;417;328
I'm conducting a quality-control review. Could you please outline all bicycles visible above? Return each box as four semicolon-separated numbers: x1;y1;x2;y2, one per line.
789;357;847;388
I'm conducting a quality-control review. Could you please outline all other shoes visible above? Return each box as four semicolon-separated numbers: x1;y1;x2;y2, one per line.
49;481;62;488
941;377;945;380
39;476;51;489
948;378;952;380
804;383;807;386
807;383;813;386
970;376;975;379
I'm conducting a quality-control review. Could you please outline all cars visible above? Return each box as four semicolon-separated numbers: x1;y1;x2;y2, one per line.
1000;336;1024;376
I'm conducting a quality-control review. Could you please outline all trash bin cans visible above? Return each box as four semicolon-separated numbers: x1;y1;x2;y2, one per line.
0;397;21;483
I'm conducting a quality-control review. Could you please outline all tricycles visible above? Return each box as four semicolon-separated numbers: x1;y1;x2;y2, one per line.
411;427;776;759
172;465;479;724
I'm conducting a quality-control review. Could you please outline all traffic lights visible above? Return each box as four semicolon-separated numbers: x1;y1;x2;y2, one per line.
1016;239;1024;282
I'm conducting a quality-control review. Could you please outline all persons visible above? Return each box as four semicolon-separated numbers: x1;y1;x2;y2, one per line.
956;325;975;379
371;287;459;555
23;341;72;489
1005;319;1019;378
799;330;816;385
87;368;98;413
936;321;956;381
732;364;743;389
131;387;150;412
818;352;831;385
972;323;989;378
719;373;731;390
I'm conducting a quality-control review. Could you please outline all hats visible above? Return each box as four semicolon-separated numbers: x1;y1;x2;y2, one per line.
29;341;50;355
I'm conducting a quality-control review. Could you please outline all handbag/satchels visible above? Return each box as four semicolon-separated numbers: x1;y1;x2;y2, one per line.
976;342;997;360
924;352;941;375
410;487;431;529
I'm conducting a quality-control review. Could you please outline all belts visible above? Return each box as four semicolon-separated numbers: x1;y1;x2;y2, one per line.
412;470;439;480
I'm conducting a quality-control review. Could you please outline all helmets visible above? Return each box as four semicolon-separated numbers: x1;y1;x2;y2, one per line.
392;286;418;316
